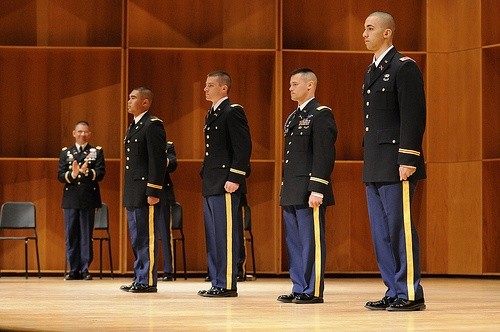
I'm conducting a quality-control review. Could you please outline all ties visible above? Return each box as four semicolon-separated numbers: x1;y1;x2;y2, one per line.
80;146;82;151
209;107;214;119
294;108;301;118
369;63;376;83
129;120;135;133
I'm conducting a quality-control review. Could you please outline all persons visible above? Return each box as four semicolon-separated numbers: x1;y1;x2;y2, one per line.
58;121;105;280
197;72;253;297
120;87;167;293
132;142;177;281
277;69;338;304
363;11;426;312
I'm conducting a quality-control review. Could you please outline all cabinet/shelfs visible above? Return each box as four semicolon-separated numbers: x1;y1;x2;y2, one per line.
0;0;428;274
480;0;500;275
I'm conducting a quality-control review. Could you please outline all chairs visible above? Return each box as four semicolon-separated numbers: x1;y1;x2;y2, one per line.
204;204;257;281
156;202;188;282
64;202;113;280
0;202;42;280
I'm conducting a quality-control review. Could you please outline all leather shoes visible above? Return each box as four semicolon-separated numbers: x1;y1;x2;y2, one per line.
198;286;237;297
120;282;157;293
66;271;93;280
365;296;426;311
277;292;324;304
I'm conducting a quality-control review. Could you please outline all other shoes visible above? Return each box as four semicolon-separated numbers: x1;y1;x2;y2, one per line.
205;275;211;281
237;275;243;280
163;274;173;281
